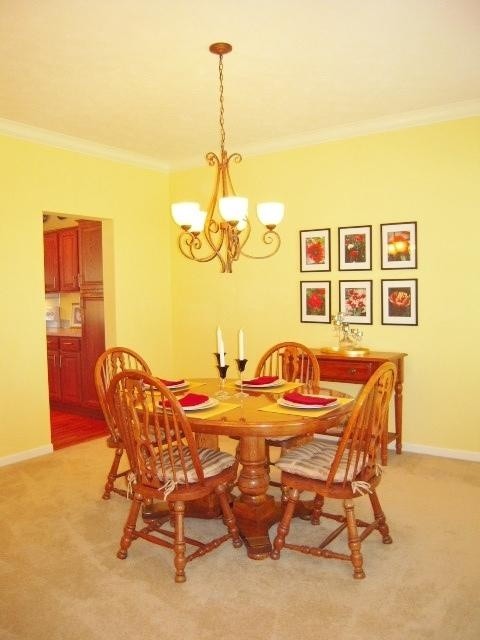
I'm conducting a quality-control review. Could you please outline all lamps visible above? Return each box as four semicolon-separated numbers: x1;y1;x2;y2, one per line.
171;43;284;273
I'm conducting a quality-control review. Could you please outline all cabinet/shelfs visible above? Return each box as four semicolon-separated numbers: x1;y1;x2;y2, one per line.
44;220;104;420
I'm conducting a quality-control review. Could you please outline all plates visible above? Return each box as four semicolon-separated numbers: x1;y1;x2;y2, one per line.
144;376;341;413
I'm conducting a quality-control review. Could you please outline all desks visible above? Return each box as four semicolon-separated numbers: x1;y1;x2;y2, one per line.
280;350;408;466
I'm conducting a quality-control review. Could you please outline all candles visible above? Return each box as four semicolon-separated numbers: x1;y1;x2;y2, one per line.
217;326;244;367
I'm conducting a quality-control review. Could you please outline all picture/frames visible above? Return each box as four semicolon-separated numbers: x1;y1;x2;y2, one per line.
300;222;418;326
